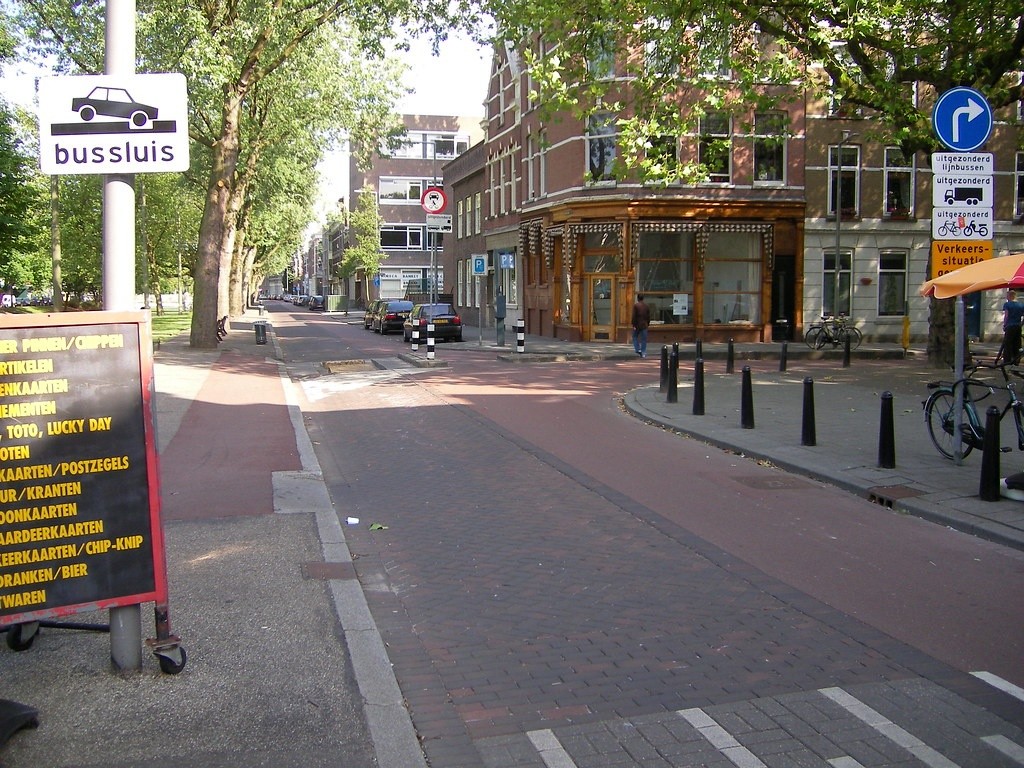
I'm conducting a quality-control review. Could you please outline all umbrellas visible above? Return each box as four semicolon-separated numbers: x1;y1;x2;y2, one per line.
919;254;1024;298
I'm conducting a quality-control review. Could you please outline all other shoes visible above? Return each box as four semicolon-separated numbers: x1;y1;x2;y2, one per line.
636;350;641;353
641;355;646;358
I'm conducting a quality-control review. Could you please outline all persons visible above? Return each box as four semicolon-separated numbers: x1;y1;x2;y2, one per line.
1002;289;1024;361
630;293;651;358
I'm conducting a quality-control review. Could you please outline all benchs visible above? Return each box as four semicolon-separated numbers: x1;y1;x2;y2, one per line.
217;315;229;341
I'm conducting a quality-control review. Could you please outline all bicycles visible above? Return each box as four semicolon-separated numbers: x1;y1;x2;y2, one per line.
921;348;1024;461
805;312;862;351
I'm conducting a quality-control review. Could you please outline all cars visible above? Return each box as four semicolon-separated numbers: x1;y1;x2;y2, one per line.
283;293;325;311
363;300;416;335
402;303;464;342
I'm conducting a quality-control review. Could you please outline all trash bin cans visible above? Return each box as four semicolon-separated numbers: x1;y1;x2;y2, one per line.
254;320;267;344
258;305;264;316
